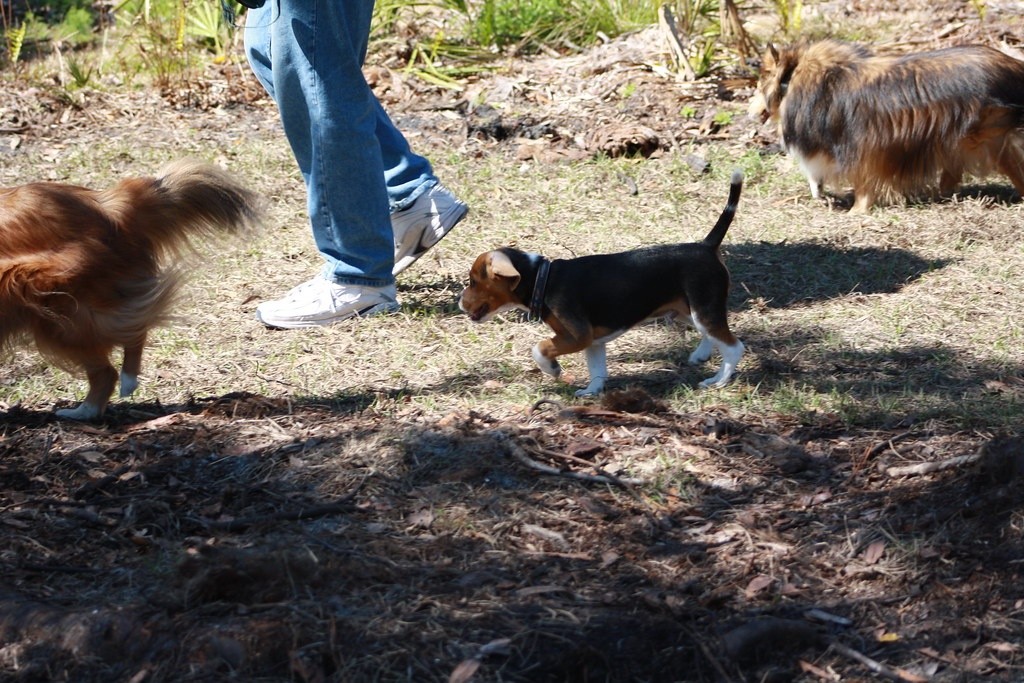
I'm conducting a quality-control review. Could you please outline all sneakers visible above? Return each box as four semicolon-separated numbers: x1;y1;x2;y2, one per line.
390;183;469;277
256;273;399;330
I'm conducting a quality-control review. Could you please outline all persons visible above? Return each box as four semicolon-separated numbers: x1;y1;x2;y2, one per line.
243;0;467;329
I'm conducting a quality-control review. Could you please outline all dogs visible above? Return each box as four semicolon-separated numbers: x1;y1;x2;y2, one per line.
744;30;1024;217
0;158;269;421
458;171;748;400
